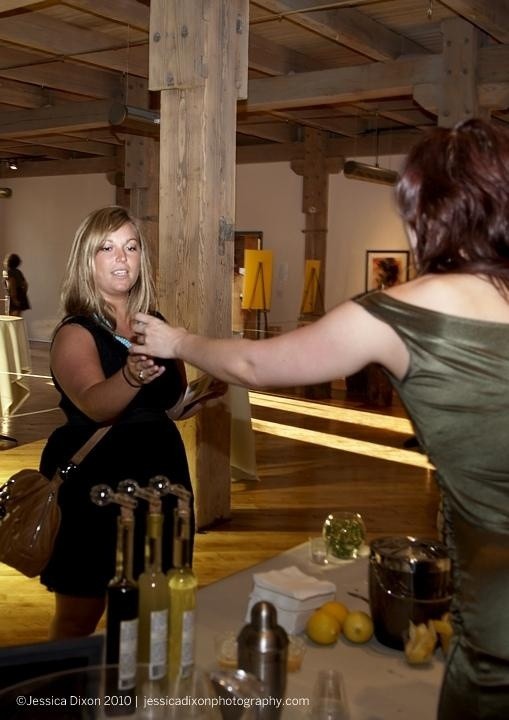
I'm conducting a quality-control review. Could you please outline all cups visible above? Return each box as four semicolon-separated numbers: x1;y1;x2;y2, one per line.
310;537;329;566
323;511;366;561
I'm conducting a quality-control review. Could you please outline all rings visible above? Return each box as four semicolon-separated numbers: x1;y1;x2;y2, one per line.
139;372;146;381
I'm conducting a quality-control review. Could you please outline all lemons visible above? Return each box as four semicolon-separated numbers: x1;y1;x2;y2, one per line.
343;611;374;643
304;611;341;645
317;601;350;632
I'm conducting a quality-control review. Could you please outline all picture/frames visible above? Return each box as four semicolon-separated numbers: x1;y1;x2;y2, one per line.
363;247;412;296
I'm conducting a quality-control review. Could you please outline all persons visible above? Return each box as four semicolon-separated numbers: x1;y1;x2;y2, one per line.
126;117;509;720
39;205;229;640
4;254;31;317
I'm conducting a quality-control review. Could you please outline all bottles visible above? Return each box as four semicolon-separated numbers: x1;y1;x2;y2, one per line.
104;518;138;696
167;511;198;679
105;697;138;720
136;513;169;681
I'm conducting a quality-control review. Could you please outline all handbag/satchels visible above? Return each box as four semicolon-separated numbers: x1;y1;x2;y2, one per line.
0;419;114;578
